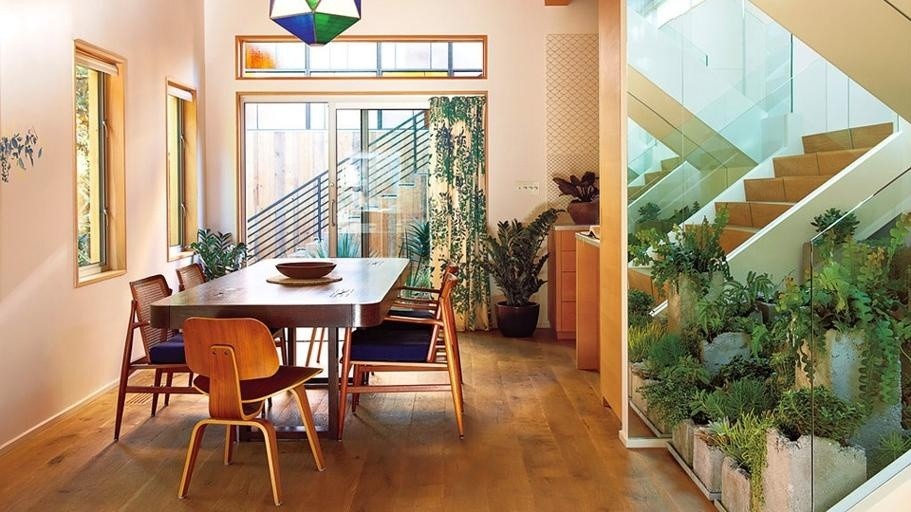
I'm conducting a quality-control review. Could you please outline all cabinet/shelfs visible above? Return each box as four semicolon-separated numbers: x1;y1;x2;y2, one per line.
548;223;578;342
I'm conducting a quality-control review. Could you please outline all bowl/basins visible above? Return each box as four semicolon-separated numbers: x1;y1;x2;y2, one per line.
276;261;337;279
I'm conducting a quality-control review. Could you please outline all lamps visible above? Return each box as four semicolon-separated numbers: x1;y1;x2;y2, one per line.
266;1;363;51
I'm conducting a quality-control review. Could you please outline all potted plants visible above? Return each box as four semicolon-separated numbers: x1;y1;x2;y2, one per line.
469;205;565;340
554;168;599;227
629;201;911;511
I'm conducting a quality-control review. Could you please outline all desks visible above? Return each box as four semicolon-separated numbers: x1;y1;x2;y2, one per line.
571;232;601;382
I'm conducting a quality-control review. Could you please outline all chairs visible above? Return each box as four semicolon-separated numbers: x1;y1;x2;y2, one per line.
337;270;466;443
160;262;204;408
178;314;326;506
393;260;459;318
114;274;200;441
